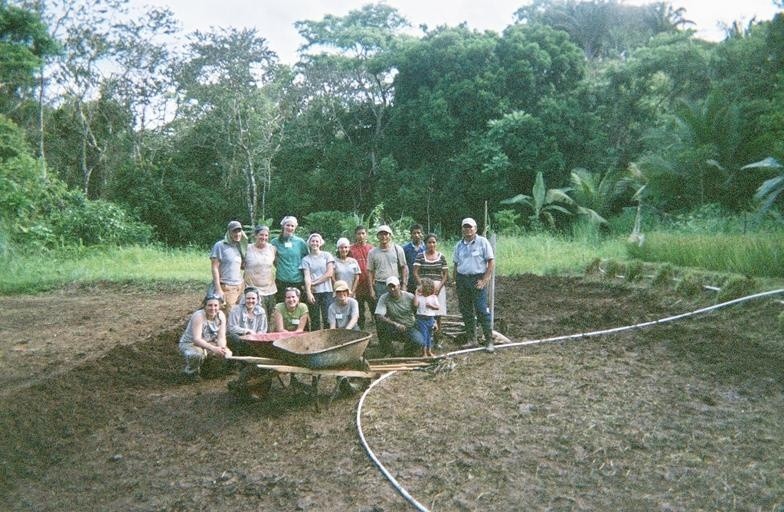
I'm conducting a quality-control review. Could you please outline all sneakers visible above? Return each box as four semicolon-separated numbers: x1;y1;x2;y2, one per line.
461;341;481;351
384;342;445;359
487;342;496;354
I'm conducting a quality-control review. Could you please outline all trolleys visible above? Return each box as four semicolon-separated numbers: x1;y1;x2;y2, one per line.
223;327;377;400
239;329;328;393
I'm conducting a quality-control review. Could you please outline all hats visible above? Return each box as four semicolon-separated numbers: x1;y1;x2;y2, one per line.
331;279;354;298
336;238;350;249
461;217;477;228
227;221;243;231
376;225;393;237
385;276;401;288
